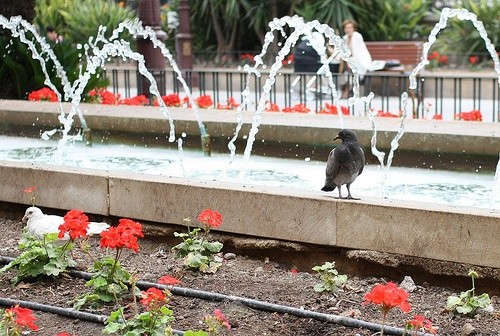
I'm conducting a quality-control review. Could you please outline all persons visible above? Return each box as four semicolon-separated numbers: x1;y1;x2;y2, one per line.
46;25;63;45
328;19;372;100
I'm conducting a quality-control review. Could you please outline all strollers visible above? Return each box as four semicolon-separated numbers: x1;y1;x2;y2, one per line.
290;34;342;102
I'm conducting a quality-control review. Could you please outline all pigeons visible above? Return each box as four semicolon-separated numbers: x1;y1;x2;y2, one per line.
22;206;111;263
321;127;366;200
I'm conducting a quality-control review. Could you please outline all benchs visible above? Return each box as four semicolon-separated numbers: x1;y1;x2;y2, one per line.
343;41;424;101
